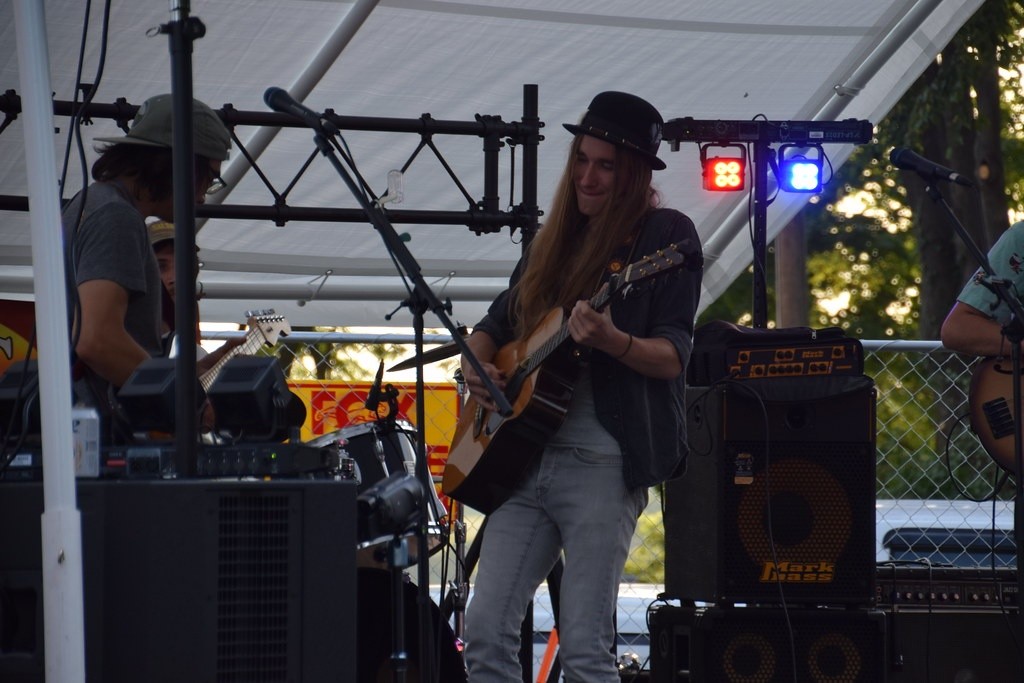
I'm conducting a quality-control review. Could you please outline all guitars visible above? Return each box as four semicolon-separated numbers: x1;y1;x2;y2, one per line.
131;305;291;446
440;238;706;518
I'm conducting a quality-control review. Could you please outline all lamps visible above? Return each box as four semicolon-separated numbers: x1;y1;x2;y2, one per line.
700;142;746;190
778;142;823;192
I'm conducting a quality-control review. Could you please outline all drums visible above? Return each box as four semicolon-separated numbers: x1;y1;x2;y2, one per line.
297;418;452;575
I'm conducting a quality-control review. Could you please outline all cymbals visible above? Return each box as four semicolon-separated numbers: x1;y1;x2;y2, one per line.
385;334;480;373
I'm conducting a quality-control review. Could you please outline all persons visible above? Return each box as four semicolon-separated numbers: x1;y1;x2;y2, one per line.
143;220;209;362
459;90;702;682
62;93;248;439
941;216;1024;359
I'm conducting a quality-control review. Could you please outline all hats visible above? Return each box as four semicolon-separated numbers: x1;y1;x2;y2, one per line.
93;94;232;160
563;91;667;169
145;220;200;252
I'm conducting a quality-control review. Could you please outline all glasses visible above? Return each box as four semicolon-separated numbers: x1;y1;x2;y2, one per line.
206;164;226;194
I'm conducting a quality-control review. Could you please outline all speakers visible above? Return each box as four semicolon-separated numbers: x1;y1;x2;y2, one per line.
650;318;1024;682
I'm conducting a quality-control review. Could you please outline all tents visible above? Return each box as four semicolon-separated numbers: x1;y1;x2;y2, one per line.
0;0;986;683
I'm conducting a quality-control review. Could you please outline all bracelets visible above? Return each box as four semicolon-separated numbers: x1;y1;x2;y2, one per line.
614;330;632;360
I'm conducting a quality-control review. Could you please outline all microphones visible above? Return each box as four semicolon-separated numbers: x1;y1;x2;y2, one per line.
890;146;974;187
264;87;342;136
